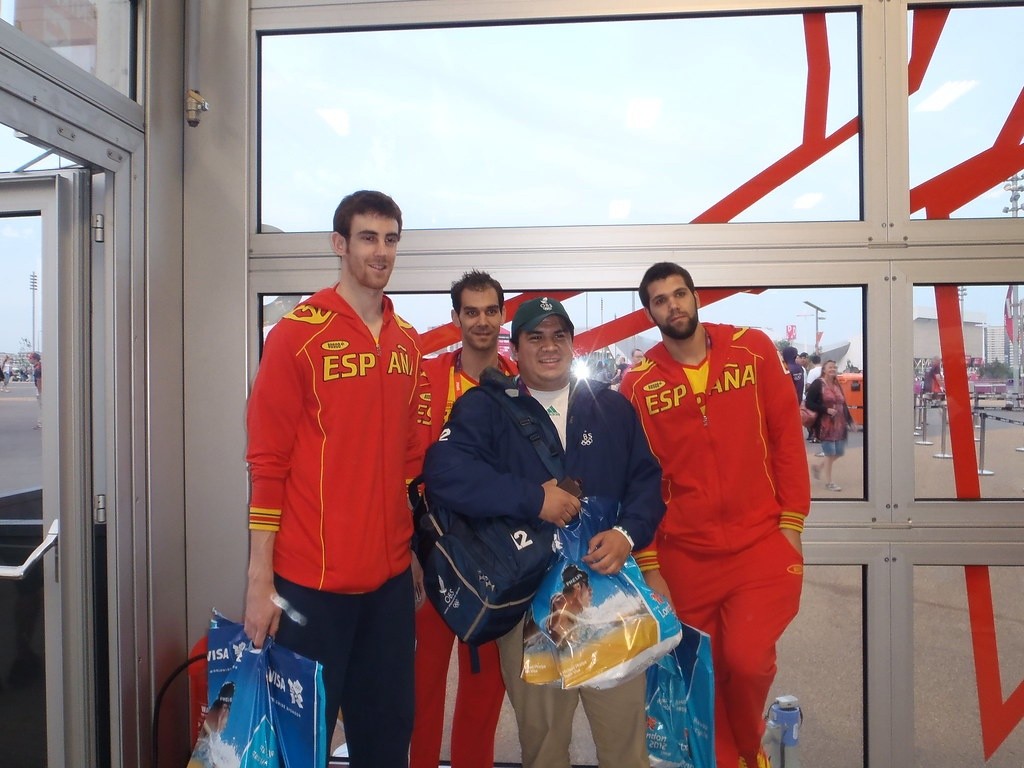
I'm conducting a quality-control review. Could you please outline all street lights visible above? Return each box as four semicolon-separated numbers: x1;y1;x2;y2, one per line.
30;270;38;354
803;300;826;352
958;285;968;324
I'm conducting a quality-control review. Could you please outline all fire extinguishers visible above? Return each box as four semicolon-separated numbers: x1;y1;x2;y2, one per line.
190;632;208;752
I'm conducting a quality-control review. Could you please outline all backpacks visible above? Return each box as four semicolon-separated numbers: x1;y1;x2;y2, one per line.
408;366;565;674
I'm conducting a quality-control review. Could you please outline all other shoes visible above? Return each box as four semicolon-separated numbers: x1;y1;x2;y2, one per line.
811;464;821;481
823;481;842;491
814;451;825;457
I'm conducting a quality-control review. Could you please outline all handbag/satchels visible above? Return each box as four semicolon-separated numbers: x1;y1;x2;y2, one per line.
644;619;717;768
187;606;326;768
519;496;683;691
799;400;818;427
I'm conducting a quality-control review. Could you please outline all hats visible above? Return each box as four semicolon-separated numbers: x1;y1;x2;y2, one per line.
783;346;800;357
511;296;574;337
799;352;809;358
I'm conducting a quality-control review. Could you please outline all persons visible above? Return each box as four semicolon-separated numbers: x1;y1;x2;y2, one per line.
11;365;32;382
0;354;12;392
544;565;593;649
618;262;811;768
409;272;520;768
421;297;668;768
805;360;858;491
199;682;235;740
237;190;423;768
27;352;41;430
588;348;643;386
781;344;822;443
923;356;944;393
